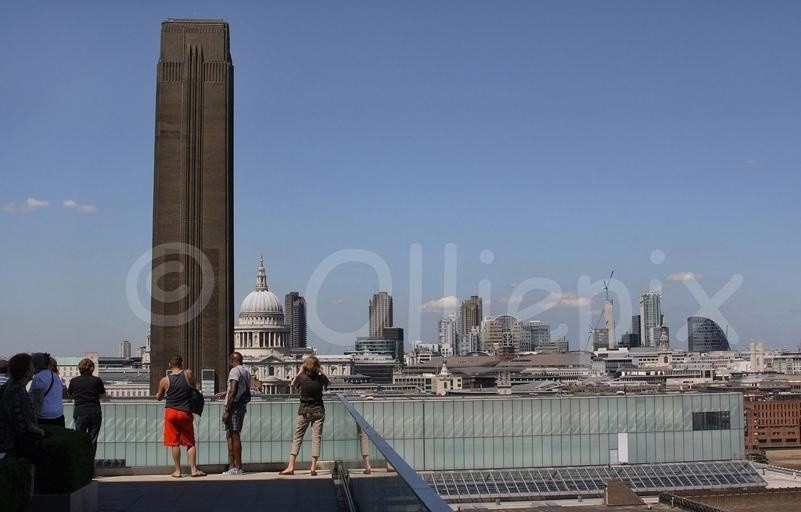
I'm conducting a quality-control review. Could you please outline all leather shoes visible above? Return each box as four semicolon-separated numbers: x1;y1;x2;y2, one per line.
387;468;395;472
311;471;317;475
279;470;294;475
364;468;371;473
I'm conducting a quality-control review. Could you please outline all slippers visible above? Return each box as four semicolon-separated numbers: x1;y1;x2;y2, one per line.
172;472;182;477
191;470;207;477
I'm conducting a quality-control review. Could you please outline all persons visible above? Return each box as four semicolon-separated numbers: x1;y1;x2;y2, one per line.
278;357;332;476
0;358;12;385
66;359;106;461
26;352;64;428
1;352;65;495
48;357;58;375
359;427;394;474
154;355;206;478
215;352;250;475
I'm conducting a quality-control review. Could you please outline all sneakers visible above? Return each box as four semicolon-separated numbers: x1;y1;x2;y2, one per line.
223;468;243;475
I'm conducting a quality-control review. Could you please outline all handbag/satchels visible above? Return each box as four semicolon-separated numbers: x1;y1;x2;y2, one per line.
190;389;204;416
232;376;251;405
